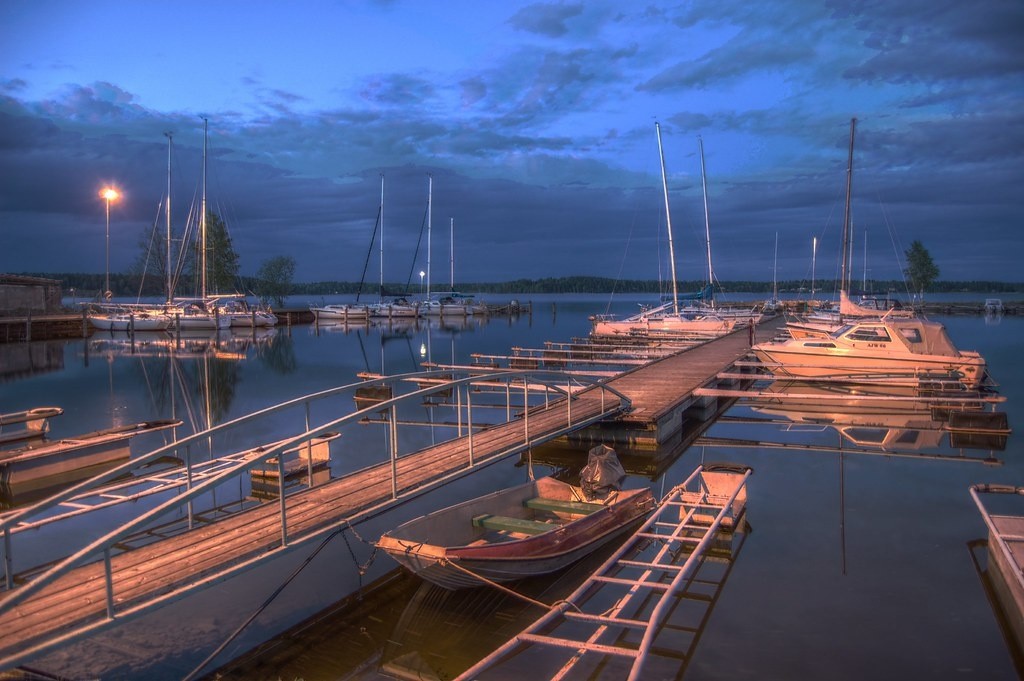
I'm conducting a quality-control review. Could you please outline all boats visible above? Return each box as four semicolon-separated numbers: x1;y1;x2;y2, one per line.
378;527;629;681
369;441;656;592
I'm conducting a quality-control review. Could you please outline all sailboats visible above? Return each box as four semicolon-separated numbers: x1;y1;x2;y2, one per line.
585;113;987;396
90;111;493;331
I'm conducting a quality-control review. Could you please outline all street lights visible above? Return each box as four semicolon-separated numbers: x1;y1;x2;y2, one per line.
103;188;118;304
